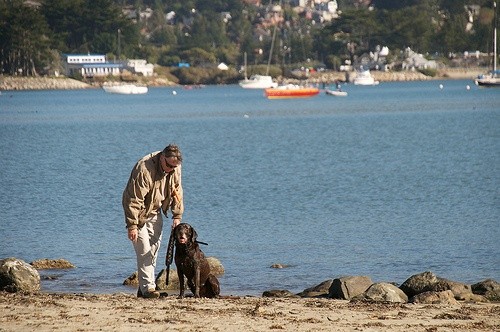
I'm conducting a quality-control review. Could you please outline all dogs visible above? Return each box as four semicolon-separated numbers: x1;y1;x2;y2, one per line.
172;223;240;300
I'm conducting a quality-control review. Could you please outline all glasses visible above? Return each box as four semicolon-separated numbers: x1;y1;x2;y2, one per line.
163;157;177;168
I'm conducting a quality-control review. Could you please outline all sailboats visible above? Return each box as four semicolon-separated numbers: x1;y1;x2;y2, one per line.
263;0;319;100
103;28;147;95
475;0;500;84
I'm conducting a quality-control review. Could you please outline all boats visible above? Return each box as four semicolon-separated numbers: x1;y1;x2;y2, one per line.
326;88;347;97
354;68;379;87
238;74;278;89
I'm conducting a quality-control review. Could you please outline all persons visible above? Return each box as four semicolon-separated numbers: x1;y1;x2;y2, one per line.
122;144;184;298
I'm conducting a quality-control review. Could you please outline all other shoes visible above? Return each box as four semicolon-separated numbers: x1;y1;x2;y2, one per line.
137;288;160;298
